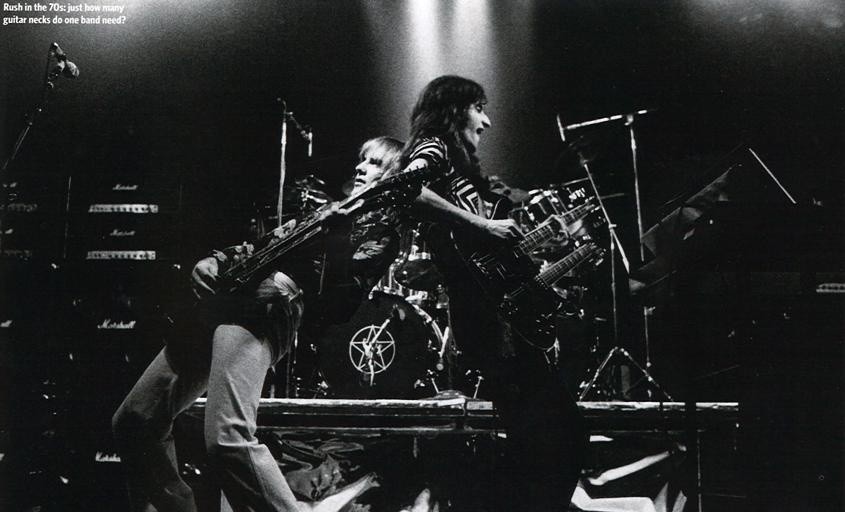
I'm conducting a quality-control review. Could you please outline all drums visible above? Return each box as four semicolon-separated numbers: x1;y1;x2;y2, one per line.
391;224;442;291
317;291;443;398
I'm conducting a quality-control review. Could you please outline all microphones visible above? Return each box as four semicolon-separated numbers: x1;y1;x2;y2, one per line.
555;108;567;143
305;123;314;155
51;40;82;80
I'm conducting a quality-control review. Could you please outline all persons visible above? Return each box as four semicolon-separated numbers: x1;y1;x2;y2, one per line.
381;74;589;511
111;136;406;512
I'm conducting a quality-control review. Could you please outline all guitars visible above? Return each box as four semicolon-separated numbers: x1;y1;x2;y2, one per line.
445;183;606;351
139;164;439;348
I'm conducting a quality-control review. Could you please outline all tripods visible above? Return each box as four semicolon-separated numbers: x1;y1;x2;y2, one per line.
573;150;677;402
